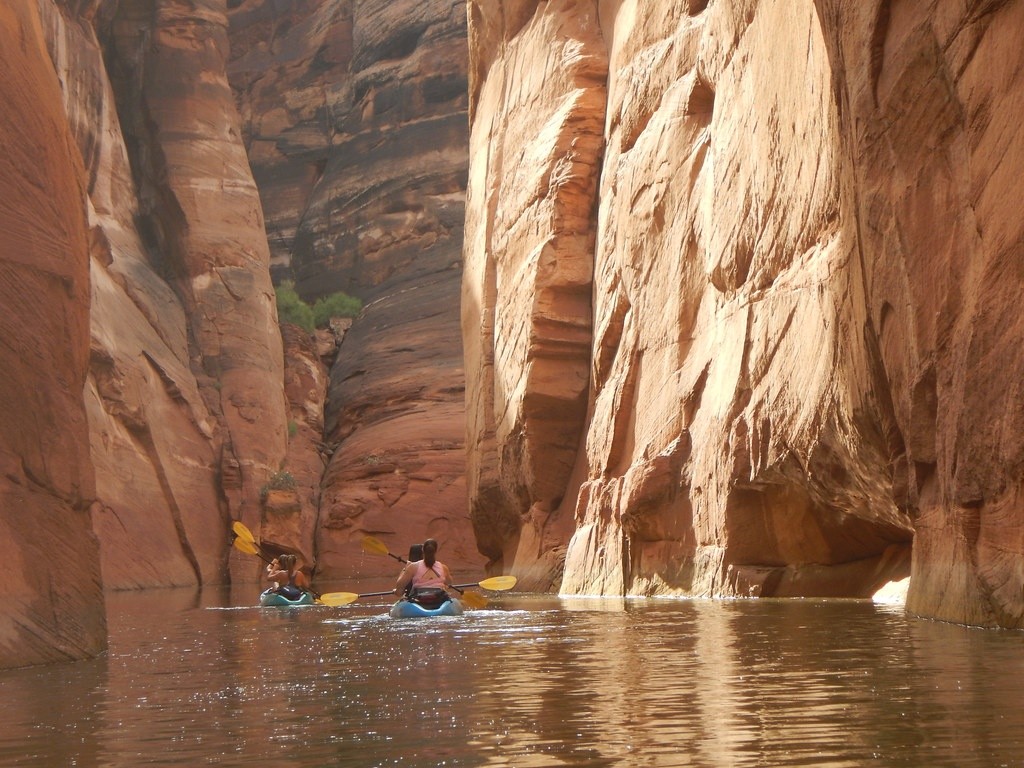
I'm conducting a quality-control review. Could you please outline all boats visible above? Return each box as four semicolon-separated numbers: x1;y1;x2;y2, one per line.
261;592;315;606
389;598;464;617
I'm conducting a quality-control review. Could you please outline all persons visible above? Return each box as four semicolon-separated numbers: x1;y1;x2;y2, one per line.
266;554;306;595
395;539;453;602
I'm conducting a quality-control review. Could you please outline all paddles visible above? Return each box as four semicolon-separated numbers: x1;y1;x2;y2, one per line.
233;536;321;603
320;575;518;607
361;535;489;609
232;520;321;598
